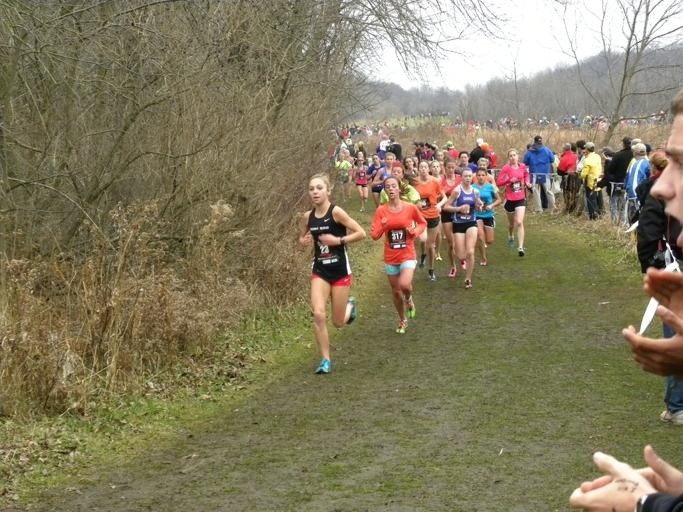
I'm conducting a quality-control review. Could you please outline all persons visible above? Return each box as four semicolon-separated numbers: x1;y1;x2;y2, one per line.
557;113;667;234
330;112;561;334
563;89;683;512
621;269;683;375
296;172;366;374
636;191;683;423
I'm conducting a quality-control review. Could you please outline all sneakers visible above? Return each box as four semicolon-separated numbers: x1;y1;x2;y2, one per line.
434;252;443;263
507;234;516;246
419;254;427;267
347;296;359;325
395;319;409;335
404;296;417;319
427;267;437;282
517;248;525;257
313;358;332;375
464;278;472;289
480;257;489;267
448;266;457;279
459;259;466;269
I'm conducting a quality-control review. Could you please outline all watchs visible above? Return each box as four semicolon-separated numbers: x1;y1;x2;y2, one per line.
340;237;347;245
633;494;648;511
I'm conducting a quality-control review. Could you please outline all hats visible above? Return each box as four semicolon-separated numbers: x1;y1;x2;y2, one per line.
631;143;647;155
480;143;489;152
442;142;454;149
623;136;633;145
583;142;595;150
